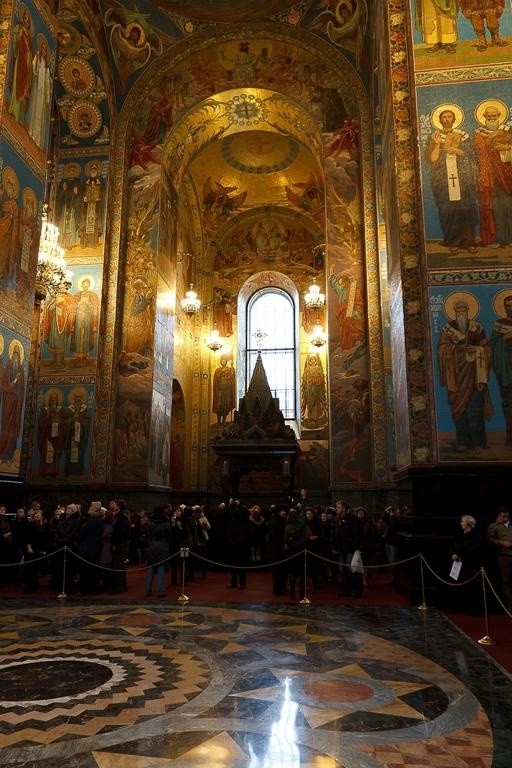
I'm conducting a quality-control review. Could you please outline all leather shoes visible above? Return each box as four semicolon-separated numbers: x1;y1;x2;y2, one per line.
144;574;308;602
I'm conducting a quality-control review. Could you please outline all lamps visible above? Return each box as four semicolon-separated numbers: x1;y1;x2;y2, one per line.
34;160;73;305
177;252;201;317
304;243;326;311
309;299;327;348
202;303;223;351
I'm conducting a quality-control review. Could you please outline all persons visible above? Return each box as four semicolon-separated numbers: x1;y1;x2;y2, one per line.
2;487;511;611
